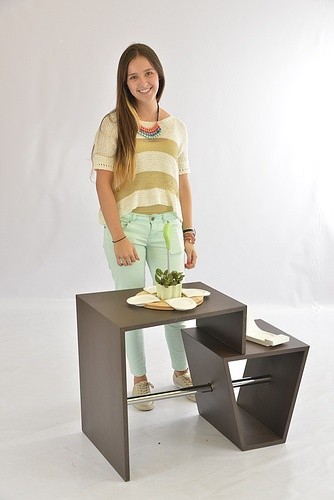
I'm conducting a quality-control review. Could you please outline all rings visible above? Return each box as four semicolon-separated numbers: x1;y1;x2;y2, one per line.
119;259;124;263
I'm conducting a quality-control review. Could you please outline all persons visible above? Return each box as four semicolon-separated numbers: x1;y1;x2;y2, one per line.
90;44;196;411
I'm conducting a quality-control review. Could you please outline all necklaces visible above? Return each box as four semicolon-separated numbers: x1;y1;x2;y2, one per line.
132;104;162;139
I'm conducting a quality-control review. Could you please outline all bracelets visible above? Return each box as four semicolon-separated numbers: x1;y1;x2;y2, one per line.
183;229;197;244
112;236;126;243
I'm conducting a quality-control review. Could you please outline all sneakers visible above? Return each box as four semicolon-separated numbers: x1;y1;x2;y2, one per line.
132;380;154;410
172;370;196;402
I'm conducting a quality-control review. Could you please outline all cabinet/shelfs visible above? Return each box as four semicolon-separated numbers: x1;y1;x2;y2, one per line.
75;281;310;482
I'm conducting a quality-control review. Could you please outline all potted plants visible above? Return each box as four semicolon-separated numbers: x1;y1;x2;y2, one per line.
154;223;185;301
